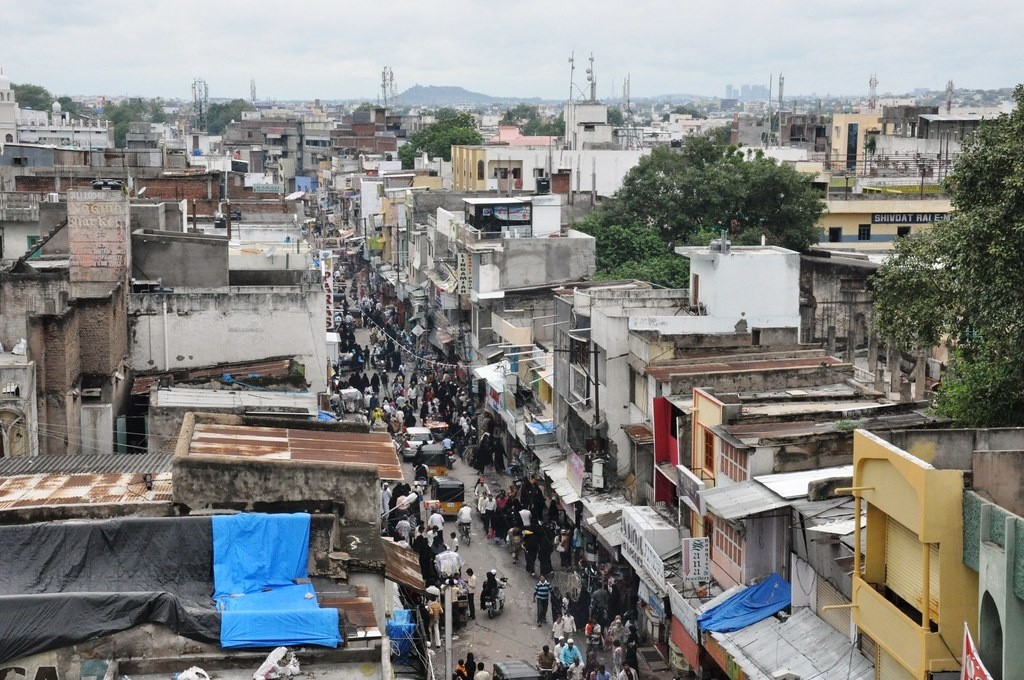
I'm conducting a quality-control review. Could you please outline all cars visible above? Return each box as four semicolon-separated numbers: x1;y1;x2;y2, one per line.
399;427;437;461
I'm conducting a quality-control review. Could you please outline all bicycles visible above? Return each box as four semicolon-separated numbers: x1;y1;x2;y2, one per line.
458;524;472;546
539;666;553;680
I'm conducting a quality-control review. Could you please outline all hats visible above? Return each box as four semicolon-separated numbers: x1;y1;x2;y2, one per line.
480;477;484;483
414;481;418;486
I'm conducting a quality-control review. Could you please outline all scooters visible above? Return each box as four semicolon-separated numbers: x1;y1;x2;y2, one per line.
371;353;378;367
485;576;508;619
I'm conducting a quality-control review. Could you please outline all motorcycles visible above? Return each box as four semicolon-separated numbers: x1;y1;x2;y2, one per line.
415;443;449;483
446;449;453;469
348;307;363;327
428;475;465;519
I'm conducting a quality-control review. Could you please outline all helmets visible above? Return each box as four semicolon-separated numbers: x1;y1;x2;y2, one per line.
491;569;497;575
567;639;574;644
559;636;564;641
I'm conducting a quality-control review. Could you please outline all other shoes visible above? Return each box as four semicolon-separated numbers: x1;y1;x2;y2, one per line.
538;621;541;626
513;560;519;564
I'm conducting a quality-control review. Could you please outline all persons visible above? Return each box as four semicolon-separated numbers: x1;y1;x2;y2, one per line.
308;217;510;476
379;458;640;680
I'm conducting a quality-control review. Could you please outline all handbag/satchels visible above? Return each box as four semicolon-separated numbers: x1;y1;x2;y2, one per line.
571;540;576;548
557;542;565;553
